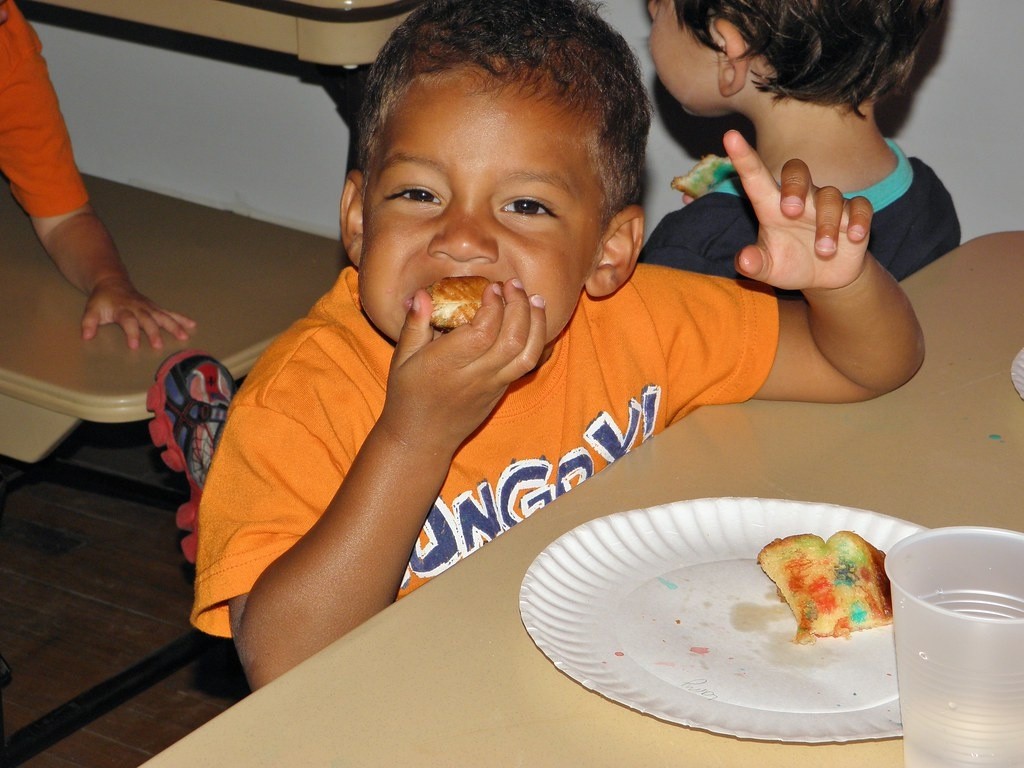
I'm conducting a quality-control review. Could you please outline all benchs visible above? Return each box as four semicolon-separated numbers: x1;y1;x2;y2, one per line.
1;172;359;465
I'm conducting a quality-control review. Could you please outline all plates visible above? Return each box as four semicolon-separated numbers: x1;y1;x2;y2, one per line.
1010;347;1024;400
518;498;928;742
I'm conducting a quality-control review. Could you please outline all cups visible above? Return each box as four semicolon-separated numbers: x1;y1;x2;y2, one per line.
885;525;1024;768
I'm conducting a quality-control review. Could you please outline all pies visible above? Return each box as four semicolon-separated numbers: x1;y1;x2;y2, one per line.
426;276;492;328
669;153;735;199
758;532;893;636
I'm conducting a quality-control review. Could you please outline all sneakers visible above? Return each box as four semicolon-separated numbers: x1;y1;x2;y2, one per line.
146;348;238;564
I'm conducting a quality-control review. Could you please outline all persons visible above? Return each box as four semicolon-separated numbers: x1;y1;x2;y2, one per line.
186;1;926;693
0;0;197;350
146;0;962;567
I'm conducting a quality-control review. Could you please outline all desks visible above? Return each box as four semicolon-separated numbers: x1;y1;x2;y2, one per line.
1;1;437;768
133;231;1024;767
